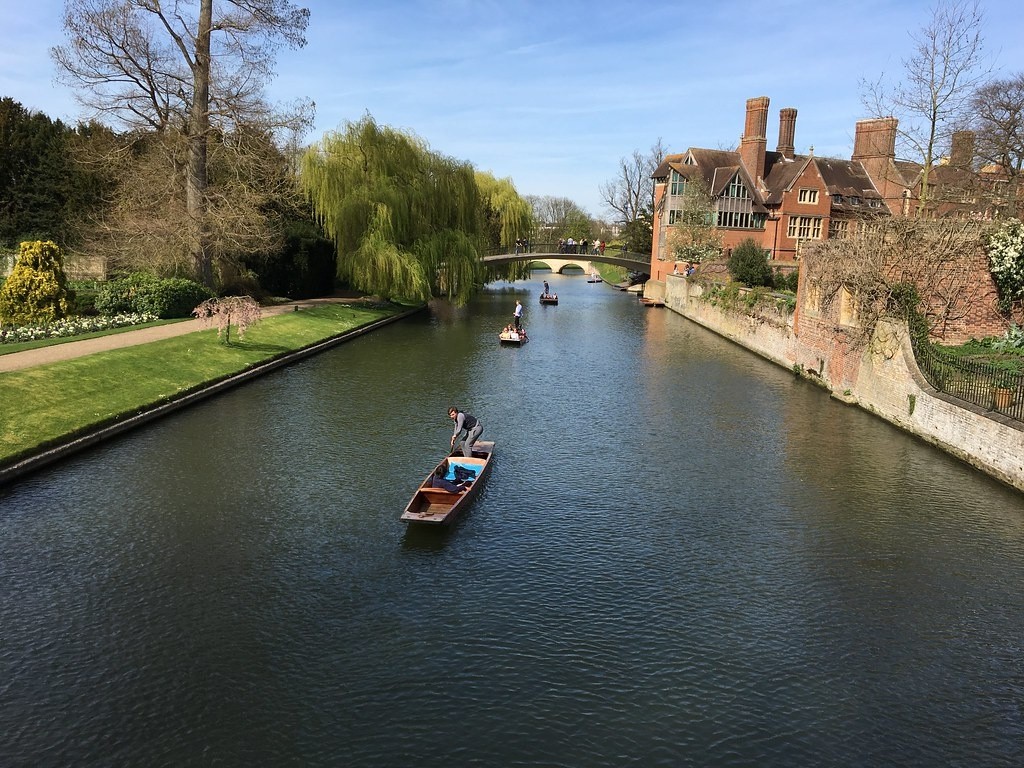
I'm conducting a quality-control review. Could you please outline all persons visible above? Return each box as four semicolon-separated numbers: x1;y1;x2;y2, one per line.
590;237;605;256
673;264;695;277
515;238;522;255
513;300;522;328
558;236;577;254
448;406;483;457
622;243;627;259
522;237;528;253
432;465;471;493
540;292;557;299
579;238;588;254
500;324;525;340
543;280;549;294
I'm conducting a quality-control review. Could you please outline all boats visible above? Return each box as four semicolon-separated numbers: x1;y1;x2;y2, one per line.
639;298;666;307
613;284;627;291
499;324;526;345
588;274;603;283
539;292;558;306
400;438;495;525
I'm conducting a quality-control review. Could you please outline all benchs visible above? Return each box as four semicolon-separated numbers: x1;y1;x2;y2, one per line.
443;462;482;482
